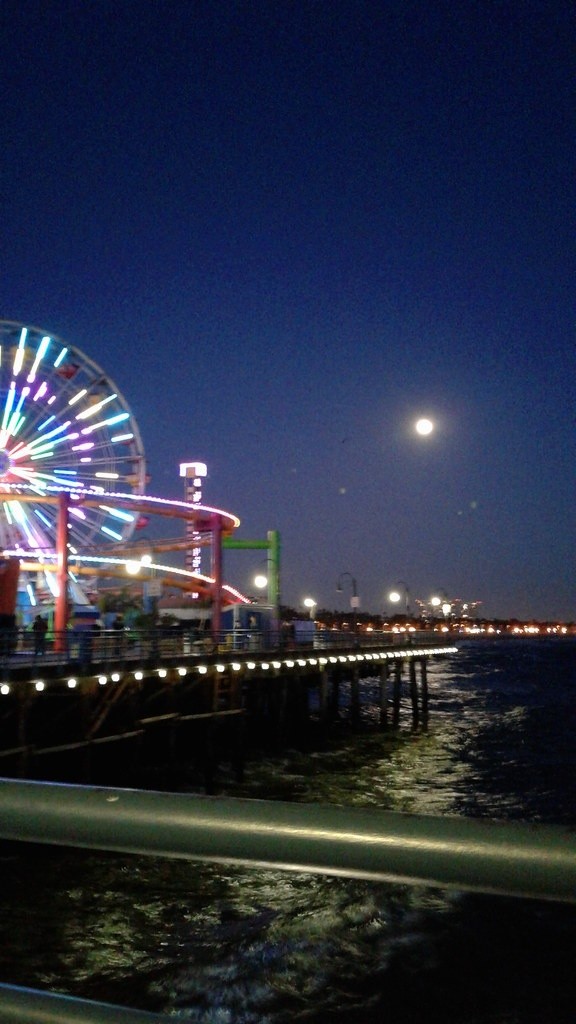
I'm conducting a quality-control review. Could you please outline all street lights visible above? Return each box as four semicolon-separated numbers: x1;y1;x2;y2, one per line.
388;581;409;621
335;571;357;632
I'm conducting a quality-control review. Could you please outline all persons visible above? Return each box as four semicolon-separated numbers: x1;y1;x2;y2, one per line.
112;615;126;659
234;616;259;649
32;615;48;656
282;616;562;646
